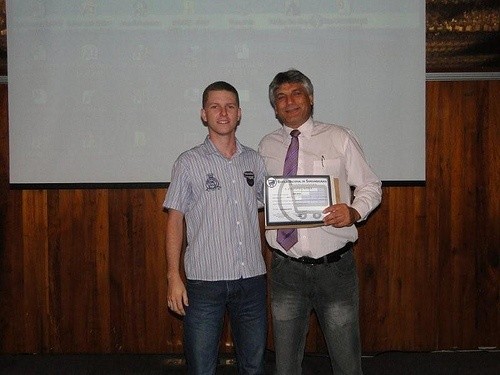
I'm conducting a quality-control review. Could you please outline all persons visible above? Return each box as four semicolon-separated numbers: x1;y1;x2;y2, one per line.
164;81;269;375
256;69;381;375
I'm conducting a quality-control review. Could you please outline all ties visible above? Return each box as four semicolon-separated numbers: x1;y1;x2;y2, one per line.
276;130;301;252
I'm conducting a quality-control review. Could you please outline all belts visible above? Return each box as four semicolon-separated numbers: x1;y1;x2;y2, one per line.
273;241;353;266
